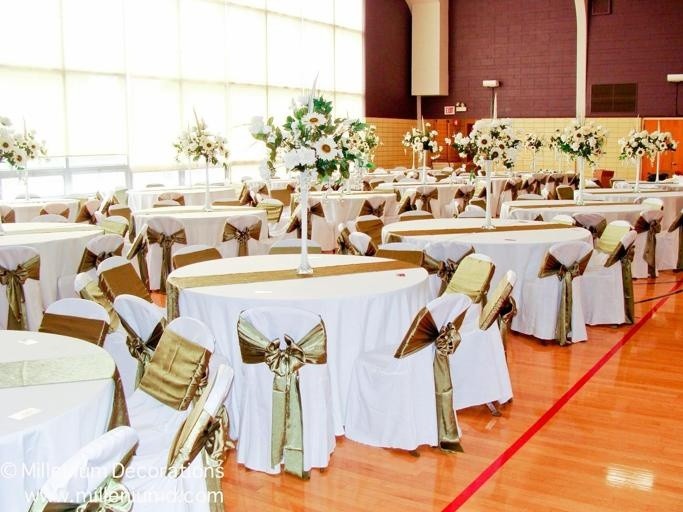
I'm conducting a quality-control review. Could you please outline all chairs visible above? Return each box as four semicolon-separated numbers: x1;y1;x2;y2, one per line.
0;168;682;512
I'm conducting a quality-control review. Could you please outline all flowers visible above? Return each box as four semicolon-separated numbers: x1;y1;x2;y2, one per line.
170;106;233;169
616;127;660;168
0;115;49;173
445;117;528;177
647;129;680;154
521;133;548;154
401;116;444;155
247;80;384;183
548;117;609;170
